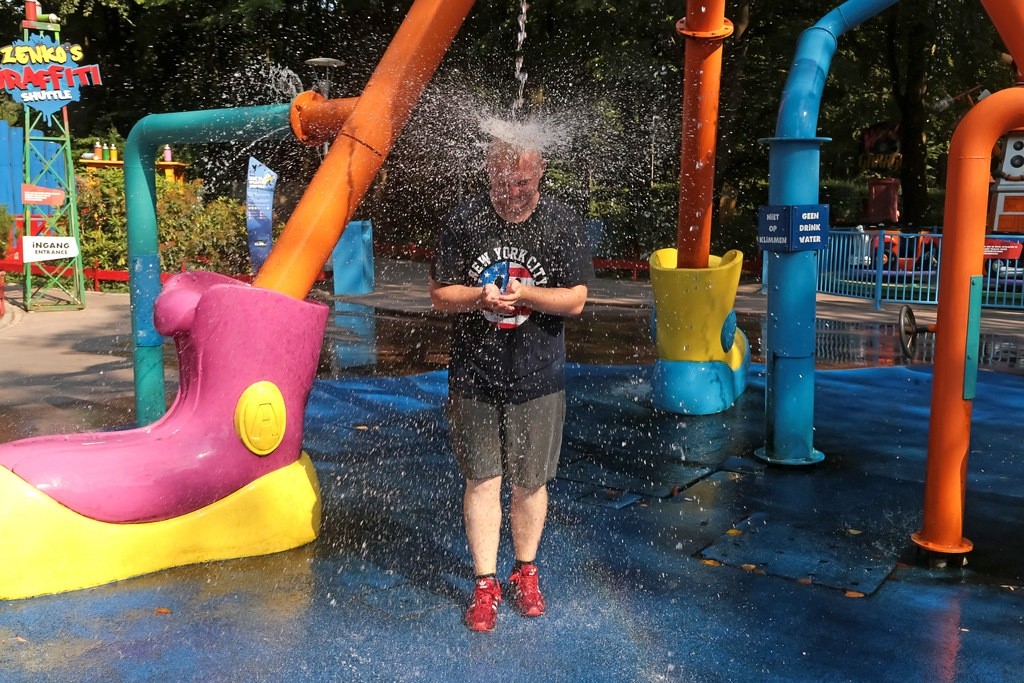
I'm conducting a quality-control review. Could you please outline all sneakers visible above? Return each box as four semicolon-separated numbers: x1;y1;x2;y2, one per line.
466;575;501;633
508;563;546;617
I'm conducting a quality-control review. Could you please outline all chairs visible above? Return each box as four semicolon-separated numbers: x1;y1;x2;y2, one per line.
866;175;901;227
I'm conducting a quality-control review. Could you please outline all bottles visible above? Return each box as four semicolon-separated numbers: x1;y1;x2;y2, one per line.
164;144;171;162
94;142;102;159
82;153;98;160
110;144;118;161
102;144;109;160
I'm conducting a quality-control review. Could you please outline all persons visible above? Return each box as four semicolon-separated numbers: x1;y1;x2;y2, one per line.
431;130;593;630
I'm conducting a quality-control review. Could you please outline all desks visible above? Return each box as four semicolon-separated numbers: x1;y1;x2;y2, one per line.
79;158;188;183
